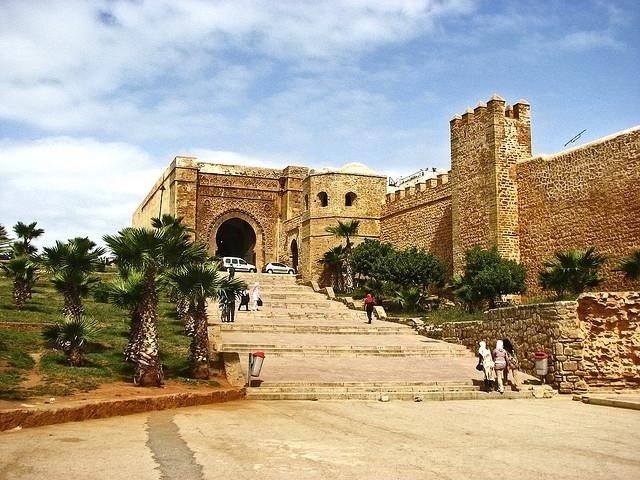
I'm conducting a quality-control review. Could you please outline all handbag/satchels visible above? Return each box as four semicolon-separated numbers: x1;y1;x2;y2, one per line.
476;365;483;370
257;298;263;306
509;356;517;369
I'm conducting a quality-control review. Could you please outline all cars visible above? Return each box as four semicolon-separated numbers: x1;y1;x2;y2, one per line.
262;262;296;274
219;257;255;272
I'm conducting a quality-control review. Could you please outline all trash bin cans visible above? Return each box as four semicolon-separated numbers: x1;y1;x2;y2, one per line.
251;350;265;377
533;350;549;378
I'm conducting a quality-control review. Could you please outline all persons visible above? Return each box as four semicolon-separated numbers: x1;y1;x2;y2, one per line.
364;294;375;324
238;288;249;311
219;293;235;322
228;263;235;281
478;338;523;394
252;282;261;311
